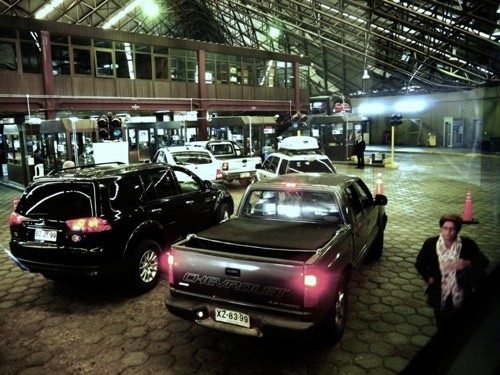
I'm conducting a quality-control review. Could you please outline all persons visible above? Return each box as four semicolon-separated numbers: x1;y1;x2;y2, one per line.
355;135;366;169
415;214;490;328
34;149;44;164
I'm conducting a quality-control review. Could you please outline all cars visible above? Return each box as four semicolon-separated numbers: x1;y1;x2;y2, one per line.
143;145;225;190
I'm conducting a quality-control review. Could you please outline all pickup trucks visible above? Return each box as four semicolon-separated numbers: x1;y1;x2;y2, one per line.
164;171;389;354
183;139;264;188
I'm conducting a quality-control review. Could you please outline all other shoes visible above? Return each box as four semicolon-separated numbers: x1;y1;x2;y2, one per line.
355;167;364;169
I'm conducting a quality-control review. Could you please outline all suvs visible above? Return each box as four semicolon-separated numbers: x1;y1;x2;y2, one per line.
8;160;235;295
252;136;338;198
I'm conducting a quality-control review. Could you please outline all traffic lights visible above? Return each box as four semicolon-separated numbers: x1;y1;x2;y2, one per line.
390;114;398;126
97;115;110;139
110;116;126;139
397;114;403;126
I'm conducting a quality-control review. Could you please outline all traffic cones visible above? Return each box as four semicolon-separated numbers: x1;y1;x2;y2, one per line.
459;190;480;225
375;170;384;196
